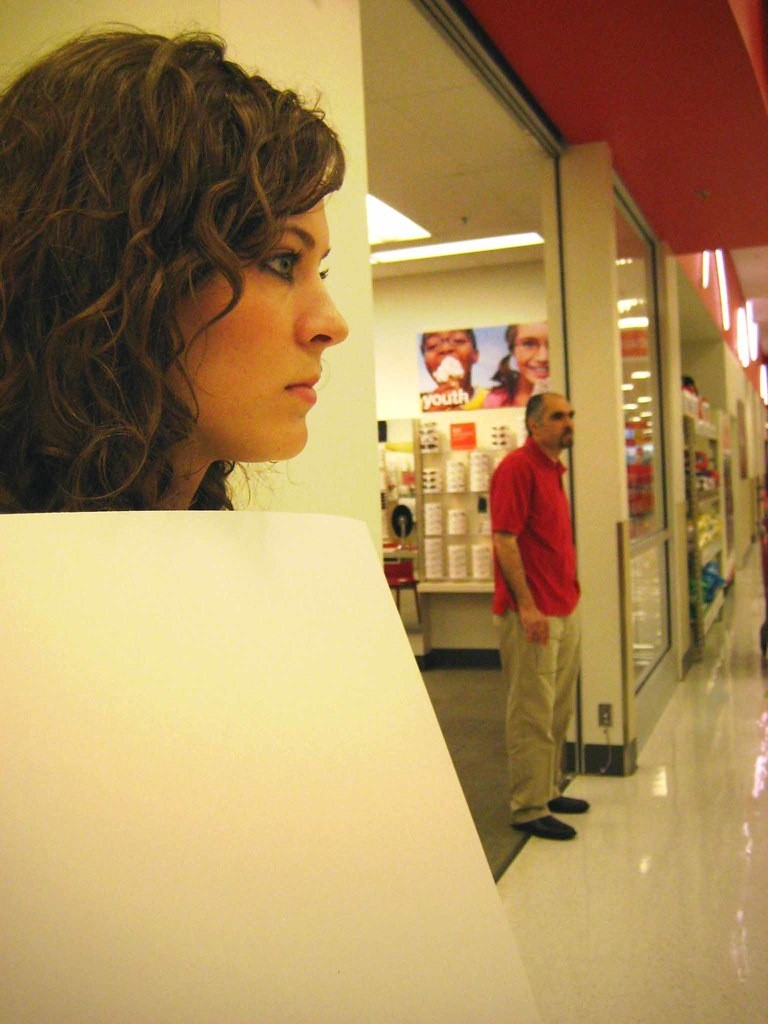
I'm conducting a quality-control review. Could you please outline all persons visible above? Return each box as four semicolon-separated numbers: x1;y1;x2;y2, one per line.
489;393;590;840
0;19;348;513
481;322;550;408
419;329;490;413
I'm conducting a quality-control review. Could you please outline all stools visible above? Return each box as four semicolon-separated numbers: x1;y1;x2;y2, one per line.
388;580;421;625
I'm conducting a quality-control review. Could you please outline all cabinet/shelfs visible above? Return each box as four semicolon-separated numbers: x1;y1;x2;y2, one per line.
377;417;510;582
683;405;729;647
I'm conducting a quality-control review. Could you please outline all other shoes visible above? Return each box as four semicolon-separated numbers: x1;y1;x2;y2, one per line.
548;797;589;814
512;815;575;839
